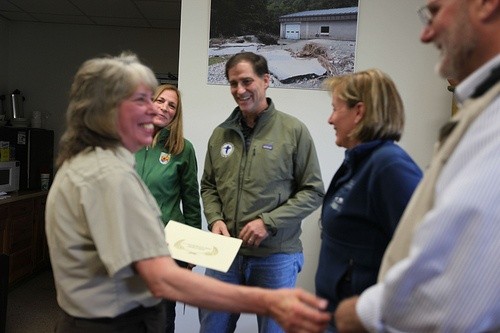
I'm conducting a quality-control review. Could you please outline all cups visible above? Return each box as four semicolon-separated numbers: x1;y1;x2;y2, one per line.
30;111;42;128
40;173;51;191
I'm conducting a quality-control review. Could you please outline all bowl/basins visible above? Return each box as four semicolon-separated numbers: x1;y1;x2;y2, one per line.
0;120;27;127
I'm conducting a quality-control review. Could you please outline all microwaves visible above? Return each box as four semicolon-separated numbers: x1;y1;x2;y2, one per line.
0;160;19;192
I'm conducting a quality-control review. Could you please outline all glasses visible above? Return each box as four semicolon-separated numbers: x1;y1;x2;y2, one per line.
417;4;435;27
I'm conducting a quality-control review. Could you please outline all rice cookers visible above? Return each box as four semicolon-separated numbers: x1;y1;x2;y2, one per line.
0;89;25;117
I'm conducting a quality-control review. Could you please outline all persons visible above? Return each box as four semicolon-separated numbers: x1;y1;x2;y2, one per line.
315;69;424;333
136;85;202;333
200;52;325;333
335;0;500;333
45;51;333;333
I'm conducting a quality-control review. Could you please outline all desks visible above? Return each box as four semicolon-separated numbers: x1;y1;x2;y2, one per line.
0;188;50;288
